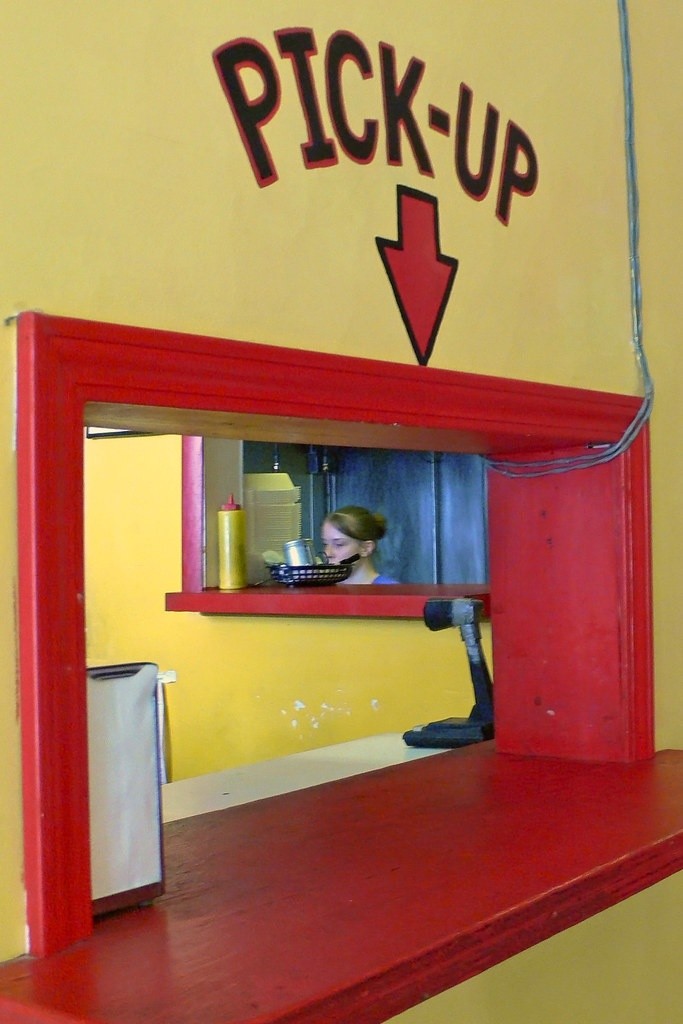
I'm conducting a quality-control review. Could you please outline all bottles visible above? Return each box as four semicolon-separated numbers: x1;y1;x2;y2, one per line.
218;494;249;590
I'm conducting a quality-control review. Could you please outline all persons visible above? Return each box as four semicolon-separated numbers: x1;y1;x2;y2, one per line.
321;506;399;584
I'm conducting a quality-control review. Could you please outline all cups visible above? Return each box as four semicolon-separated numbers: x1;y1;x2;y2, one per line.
285;539;313;567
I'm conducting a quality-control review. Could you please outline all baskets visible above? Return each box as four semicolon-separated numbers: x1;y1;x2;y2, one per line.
264;563;353;587
